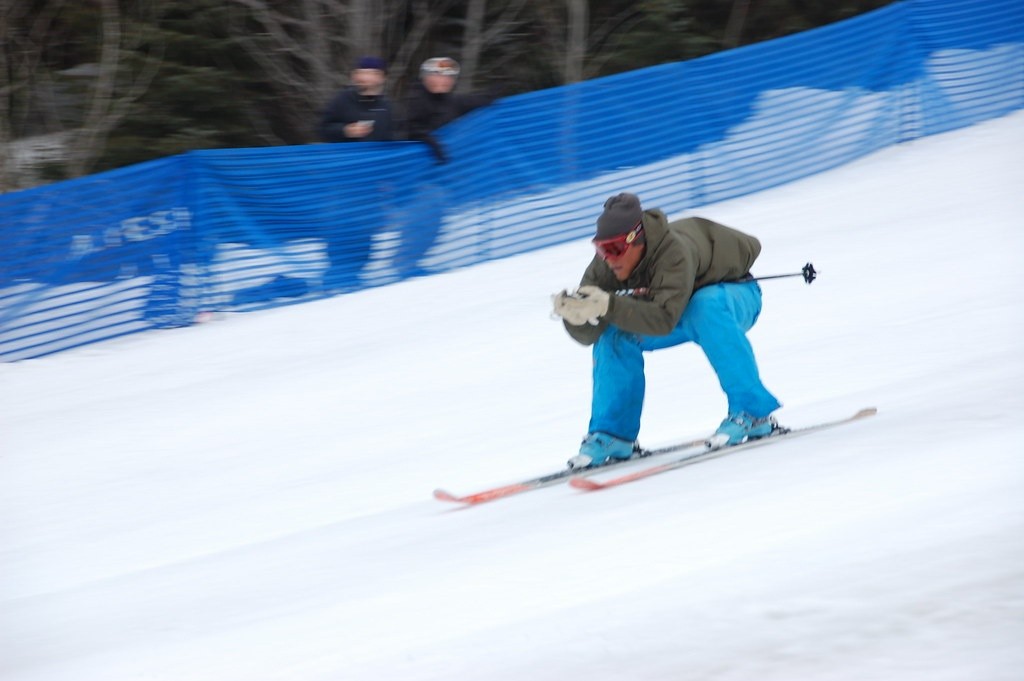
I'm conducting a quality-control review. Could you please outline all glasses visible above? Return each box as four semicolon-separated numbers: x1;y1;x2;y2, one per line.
591;235;630;261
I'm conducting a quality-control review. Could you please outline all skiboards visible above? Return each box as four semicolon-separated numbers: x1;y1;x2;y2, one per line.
433;407;878;505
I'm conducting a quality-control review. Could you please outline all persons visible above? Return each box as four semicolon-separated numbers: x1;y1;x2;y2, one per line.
549;194;782;467
395;57;494;275
312;55;402;288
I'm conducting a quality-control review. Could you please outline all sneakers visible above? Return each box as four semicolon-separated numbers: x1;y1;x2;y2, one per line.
567;431;635;472
703;411;773;450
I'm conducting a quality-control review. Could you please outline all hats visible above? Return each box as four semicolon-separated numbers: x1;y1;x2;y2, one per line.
421;58;461;76
591;193;642;244
349;56;386;71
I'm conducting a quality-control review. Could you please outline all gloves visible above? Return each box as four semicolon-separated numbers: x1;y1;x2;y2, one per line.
554;285;610;326
